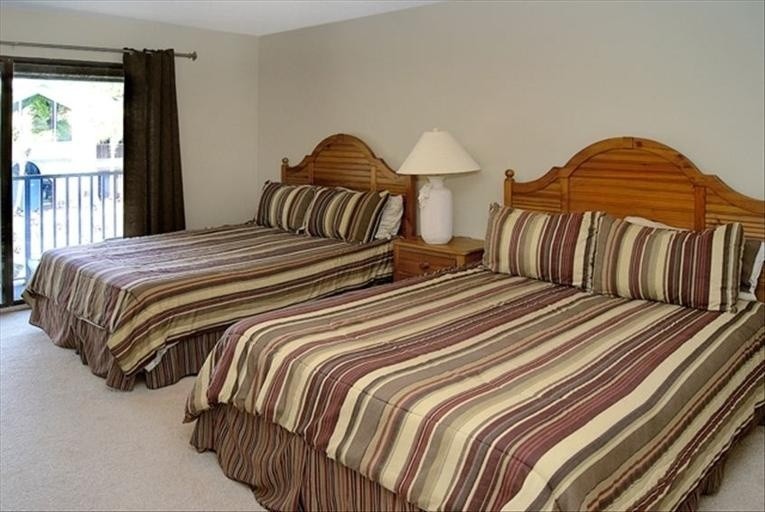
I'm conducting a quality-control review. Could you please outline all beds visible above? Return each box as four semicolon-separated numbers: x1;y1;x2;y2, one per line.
25;133;419;390
186;137;765;512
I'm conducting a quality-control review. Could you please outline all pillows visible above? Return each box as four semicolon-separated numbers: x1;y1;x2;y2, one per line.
310;186;389;245
375;192;406;239
255;180;315;234
593;205;743;312
483;199;595;291
621;212;764;303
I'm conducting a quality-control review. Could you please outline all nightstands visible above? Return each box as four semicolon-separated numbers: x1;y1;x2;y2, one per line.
387;236;485;282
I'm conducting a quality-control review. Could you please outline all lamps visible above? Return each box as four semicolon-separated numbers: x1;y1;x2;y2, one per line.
394;127;481;248
